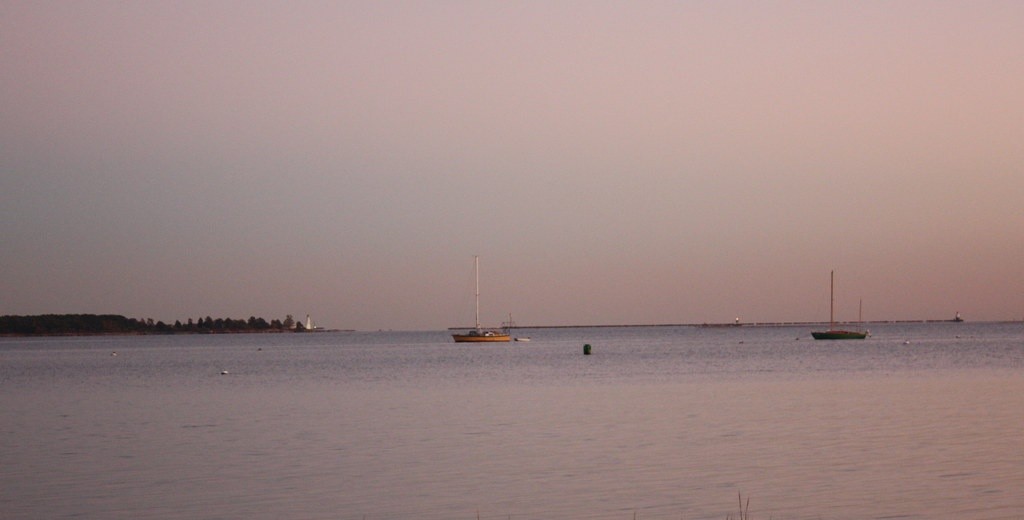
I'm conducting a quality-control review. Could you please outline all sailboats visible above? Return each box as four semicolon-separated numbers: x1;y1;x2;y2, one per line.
451;256;511;342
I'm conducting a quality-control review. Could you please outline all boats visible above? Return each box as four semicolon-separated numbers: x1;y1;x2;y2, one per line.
515;338;530;342
810;270;868;340
